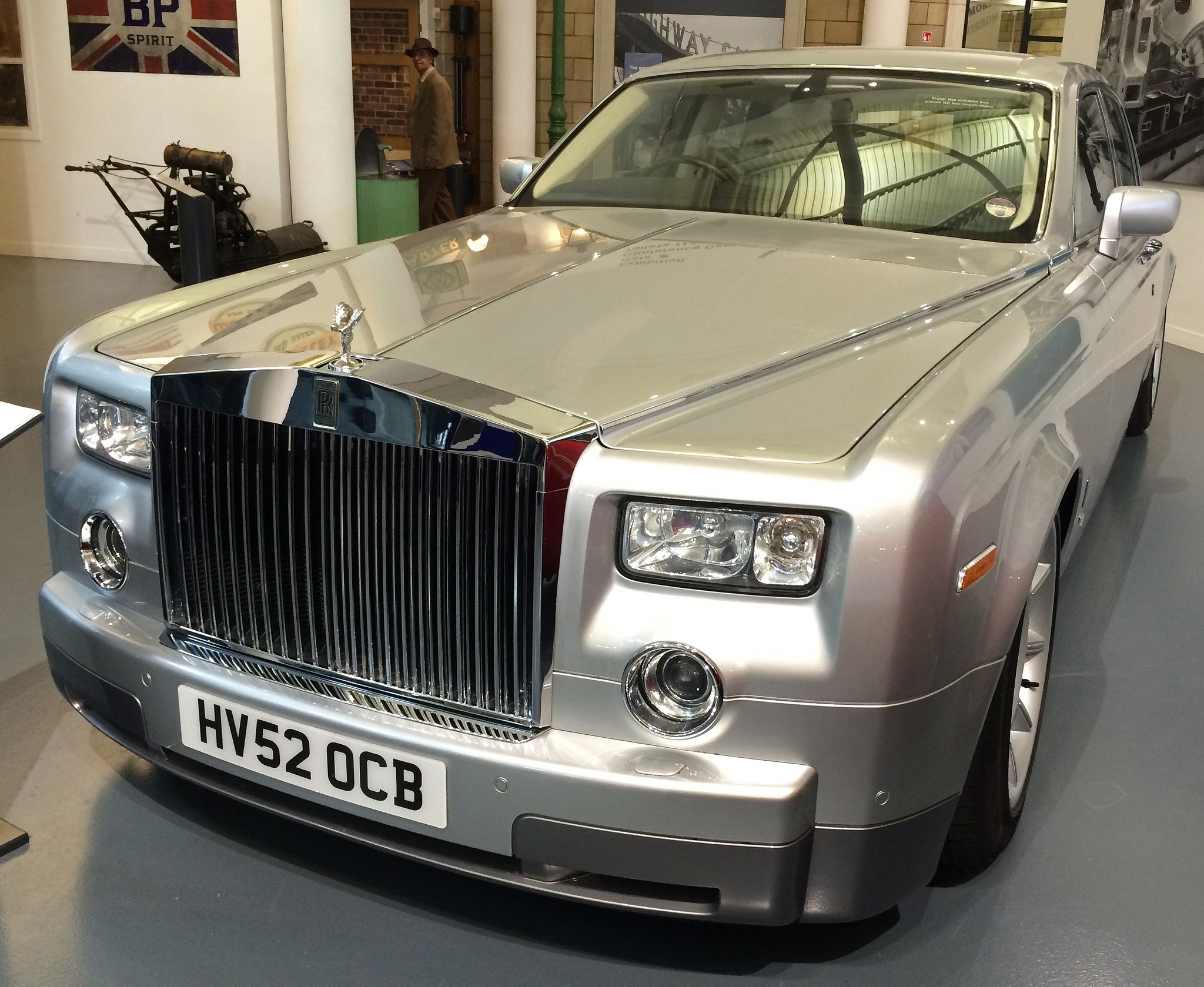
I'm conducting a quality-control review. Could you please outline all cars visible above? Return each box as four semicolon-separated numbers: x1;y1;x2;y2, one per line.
34;46;1182;931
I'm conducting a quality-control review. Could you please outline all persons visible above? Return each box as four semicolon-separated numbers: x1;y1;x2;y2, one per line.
404;37;465;229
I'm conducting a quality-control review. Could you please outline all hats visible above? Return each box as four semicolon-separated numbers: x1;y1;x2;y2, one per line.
405;38;440;57
